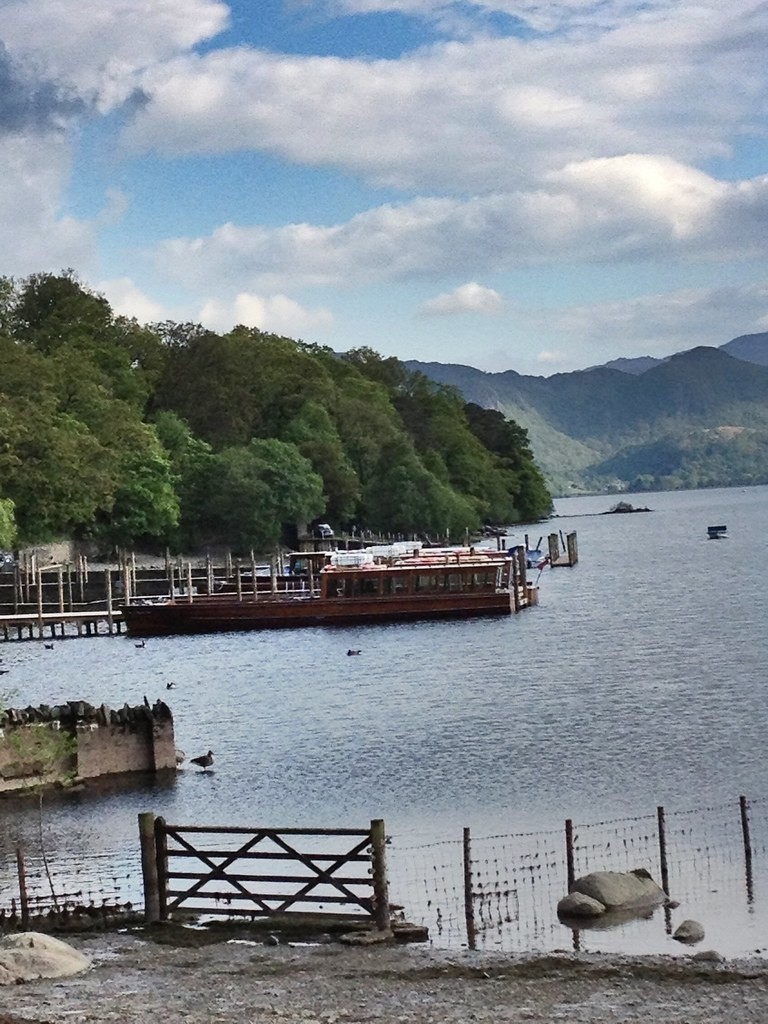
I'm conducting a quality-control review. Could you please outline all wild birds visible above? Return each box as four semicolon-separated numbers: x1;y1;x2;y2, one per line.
189;750;216;770
43;642;55;650
167;681;176;689
347;649;362;656
134;639;147;648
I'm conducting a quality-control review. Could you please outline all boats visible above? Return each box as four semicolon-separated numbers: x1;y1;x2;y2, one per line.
116;560;516;636
706;524;728;539
240;540;546;587
